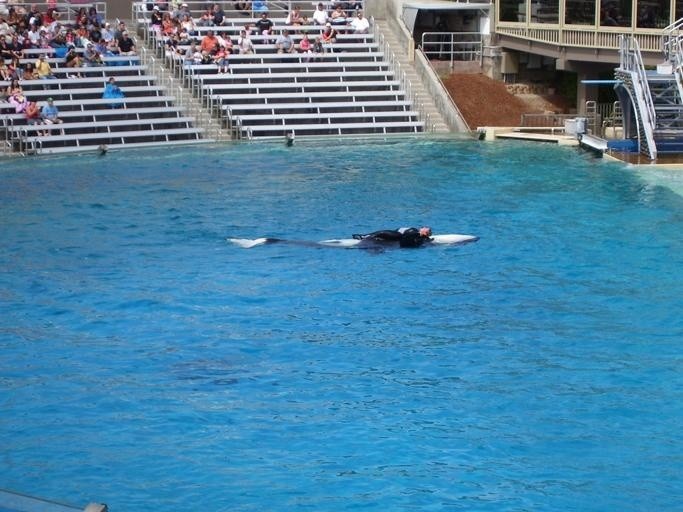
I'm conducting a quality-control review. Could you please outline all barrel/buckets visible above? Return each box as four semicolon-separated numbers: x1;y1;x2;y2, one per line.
657;63;673;75
564;119;577;134
575;118;588;134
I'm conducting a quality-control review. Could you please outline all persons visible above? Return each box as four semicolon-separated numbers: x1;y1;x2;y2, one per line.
436;17;450;58
146;0;370;74
352;226;432;248
0;0;137;136
564;0;653;28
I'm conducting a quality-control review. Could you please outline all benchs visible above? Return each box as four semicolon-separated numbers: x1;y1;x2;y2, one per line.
0;16;425;156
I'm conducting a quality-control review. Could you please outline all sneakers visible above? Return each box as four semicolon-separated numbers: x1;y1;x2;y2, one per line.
217;72;229;75
38;133;49;136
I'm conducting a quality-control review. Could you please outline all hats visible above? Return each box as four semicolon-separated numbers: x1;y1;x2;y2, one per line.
326;23;331;27
47;97;54;101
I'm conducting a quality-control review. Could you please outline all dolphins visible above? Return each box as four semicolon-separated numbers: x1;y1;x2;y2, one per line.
226;232;481;249
